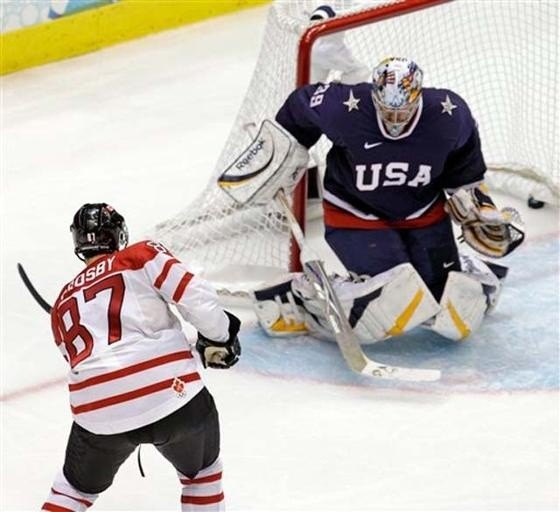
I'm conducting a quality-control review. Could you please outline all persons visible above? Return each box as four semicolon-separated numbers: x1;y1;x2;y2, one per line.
41;203;242;511
217;57;529;348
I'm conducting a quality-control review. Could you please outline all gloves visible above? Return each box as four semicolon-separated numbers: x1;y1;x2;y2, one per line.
195;311;242;370
217;116;310;212
441;182;525;257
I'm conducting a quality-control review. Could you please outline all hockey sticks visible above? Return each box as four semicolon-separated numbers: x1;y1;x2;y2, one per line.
245;121;441;383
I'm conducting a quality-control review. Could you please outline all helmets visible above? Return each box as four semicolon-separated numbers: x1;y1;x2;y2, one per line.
69;202;129;261
368;57;425;122
